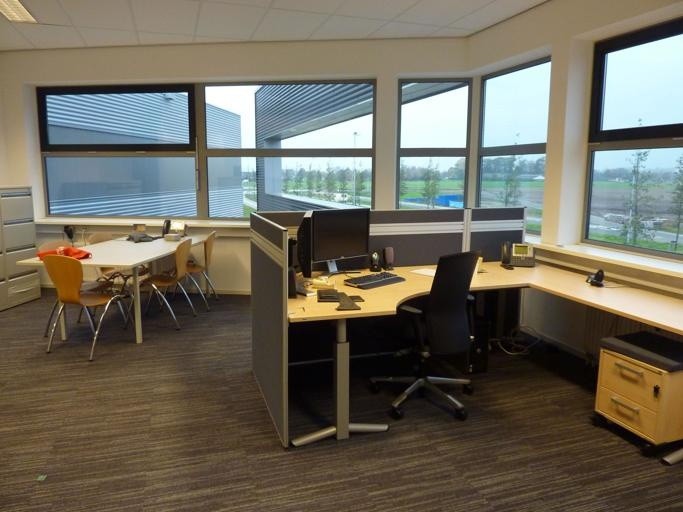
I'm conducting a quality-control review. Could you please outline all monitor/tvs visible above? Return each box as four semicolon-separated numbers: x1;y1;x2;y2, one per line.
312;208;371;279
288;210;328;298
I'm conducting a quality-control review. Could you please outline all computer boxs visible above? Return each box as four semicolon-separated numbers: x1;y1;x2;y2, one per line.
470;316;489;374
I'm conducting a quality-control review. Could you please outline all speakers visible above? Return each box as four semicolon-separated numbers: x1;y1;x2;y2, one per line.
369;249;382;272
383;246;395;271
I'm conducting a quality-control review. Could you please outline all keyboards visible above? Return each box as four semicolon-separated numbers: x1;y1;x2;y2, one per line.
343;271;406;290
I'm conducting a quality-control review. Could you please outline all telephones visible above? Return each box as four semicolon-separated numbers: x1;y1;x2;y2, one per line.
501;240;535;267
162;220;187;237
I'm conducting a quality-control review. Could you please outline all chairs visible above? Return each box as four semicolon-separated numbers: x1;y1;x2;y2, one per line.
17;231;220;362
366;249;481;421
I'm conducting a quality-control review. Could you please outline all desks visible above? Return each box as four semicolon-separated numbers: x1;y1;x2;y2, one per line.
287;260;683;466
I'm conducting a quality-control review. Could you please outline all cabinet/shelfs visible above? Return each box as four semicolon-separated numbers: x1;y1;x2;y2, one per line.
0;184;42;313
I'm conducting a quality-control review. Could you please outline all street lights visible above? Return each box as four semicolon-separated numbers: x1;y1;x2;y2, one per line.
352;132;357;207
